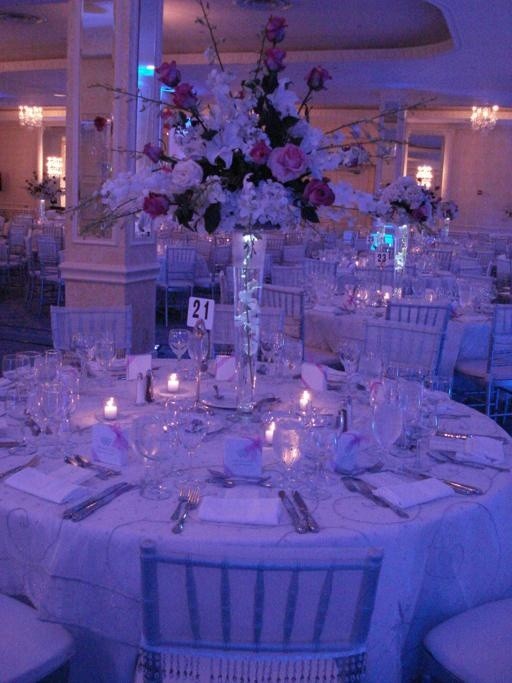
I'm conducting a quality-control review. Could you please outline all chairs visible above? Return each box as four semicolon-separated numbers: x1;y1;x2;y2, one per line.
302;258;337;292
284;231;302;245
0;593;78;683
262;287;341;369
24;236;56;312
476;251;496;270
42;223;63;250
13;212;33;228
356;320;446;392
386;299;452;376
270;266;306;287
280;245;307;266
352;268;395;294
495;257;512;279
208;305;286;359
452;307;512;417
354;238;368;252
1;245;25;298
35;233;65;311
8;224;27;255
50;304;133;355
134;539;386;683
156;247;197;328
418;596;512;683
426;249;453;271
266;238;286;265
489;233;512;276
194;246;231;300
319;231;338;250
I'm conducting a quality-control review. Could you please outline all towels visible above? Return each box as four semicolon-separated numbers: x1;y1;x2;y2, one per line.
198;495;283;526
371;478;456;509
455;436;505;464
4;466;86;504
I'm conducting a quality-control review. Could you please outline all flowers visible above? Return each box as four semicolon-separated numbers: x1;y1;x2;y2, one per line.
378;176;433;226
59;1;458;396
48;175;71;200
414;200;459;237
25;171;66;200
440;200;459;220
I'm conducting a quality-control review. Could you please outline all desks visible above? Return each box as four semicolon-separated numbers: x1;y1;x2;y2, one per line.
284;288;493;371
0;358;512;683
336;269;459;302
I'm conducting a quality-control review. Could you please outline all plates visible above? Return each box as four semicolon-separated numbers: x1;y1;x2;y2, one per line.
206;417;224;435
198;389;236;410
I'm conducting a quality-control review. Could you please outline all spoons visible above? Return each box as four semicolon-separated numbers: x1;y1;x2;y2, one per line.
341;461;384;480
208;469;275;489
65;454;119;478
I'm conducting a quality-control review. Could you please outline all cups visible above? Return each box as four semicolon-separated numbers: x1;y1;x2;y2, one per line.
2;349;81;455
262;331;302;391
371;362;452;465
73;333;95;382
189;333;210;375
96;341;117;387
165;397;188;451
168;328;192;374
135;415;175;501
304;428;336;491
176;403;207;492
271;422;300;489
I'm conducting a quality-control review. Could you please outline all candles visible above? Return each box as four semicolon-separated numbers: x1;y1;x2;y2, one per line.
384;297;389;304
264;430;273;446
104;405;119;421
167;380;179;392
299;399;309;411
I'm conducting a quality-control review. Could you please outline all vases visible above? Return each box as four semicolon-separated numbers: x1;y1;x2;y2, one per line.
393;222;411;272
49;197;58;206
441;216;450;233
39;199;45;221
424;234;436;244
231;230;268;414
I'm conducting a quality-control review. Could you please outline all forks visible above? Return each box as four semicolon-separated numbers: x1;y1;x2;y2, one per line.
346;478;411;520
170;486;189;521
2;453;44;480
172;490;202;534
426;449;510;472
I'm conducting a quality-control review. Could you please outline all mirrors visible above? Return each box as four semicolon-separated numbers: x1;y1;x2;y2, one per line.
71;0;124;247
406;135;445;198
43;126;66;208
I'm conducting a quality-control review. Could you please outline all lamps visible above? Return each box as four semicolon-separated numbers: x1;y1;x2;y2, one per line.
416;164;433;187
233;0;293;14
45;155;63;176
18;104;43;128
0;12;48;25
470;104;499;132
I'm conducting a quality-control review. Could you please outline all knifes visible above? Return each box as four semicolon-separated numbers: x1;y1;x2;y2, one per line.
292;489;321;532
400;468;483;496
277;490;306;534
63;480;135;522
438;431;509;443
